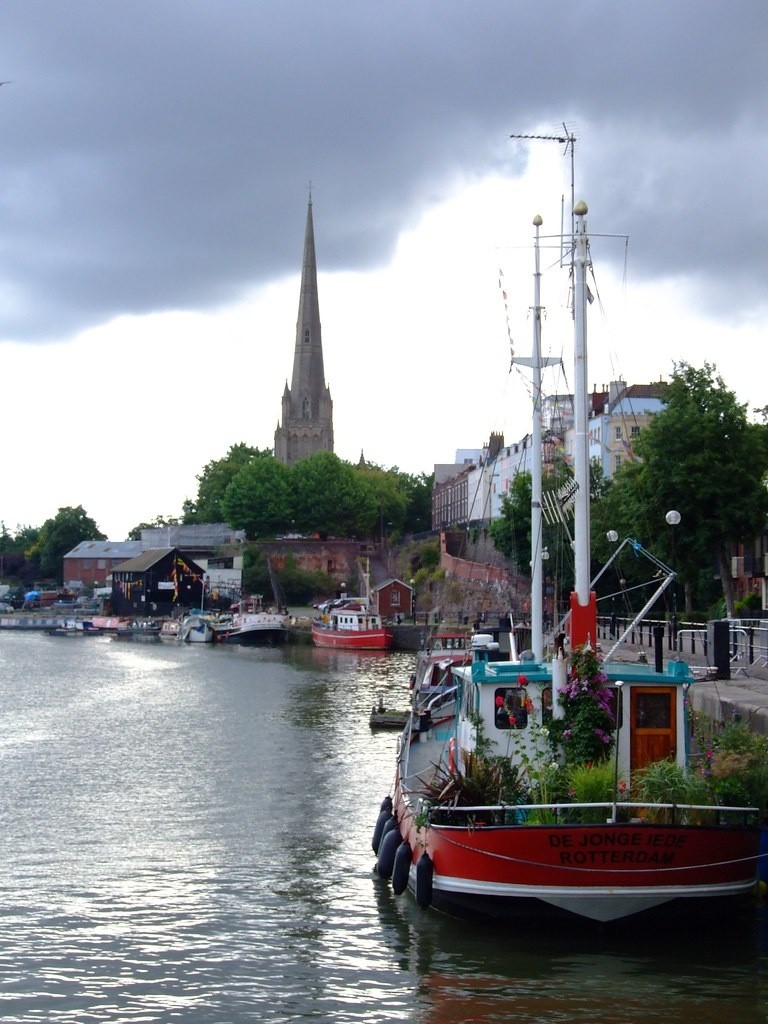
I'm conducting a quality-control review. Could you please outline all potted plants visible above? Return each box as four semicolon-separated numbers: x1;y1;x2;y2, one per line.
622;756;711;825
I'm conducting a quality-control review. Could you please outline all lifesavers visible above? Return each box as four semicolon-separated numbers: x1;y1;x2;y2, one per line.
447;737;456;773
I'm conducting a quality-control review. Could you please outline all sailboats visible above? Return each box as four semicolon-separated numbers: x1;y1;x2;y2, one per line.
373;119;763;948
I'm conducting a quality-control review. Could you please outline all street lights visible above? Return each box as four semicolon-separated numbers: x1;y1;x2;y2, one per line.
542;552;550;632
607;530;618;640
666;510;682;650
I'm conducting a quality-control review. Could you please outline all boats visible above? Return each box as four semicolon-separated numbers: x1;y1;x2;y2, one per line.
176;583;212;643
219;593;289;645
412;632;478;707
159;620;181;641
311;584;392;650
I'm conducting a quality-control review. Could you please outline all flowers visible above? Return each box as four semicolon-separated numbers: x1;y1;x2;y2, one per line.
400;630;768;850
